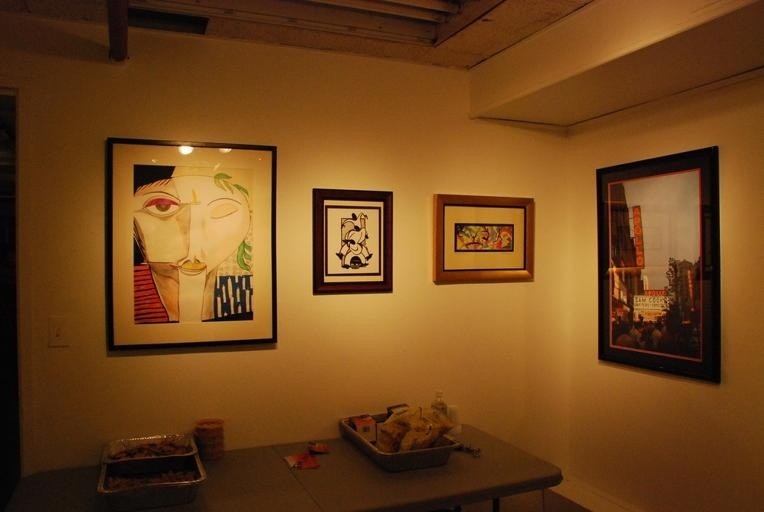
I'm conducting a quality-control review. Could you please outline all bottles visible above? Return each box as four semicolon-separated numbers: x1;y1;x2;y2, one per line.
431;391;447;421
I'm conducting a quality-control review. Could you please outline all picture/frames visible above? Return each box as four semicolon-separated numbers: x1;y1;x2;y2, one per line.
595;145;722;383
103;136;278;353
432;193;535;286
311;187;393;295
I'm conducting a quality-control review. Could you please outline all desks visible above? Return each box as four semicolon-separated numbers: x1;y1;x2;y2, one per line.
272;422;563;510
18;444;318;511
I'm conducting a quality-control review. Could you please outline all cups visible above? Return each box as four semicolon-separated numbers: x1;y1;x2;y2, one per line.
193;419;225;462
